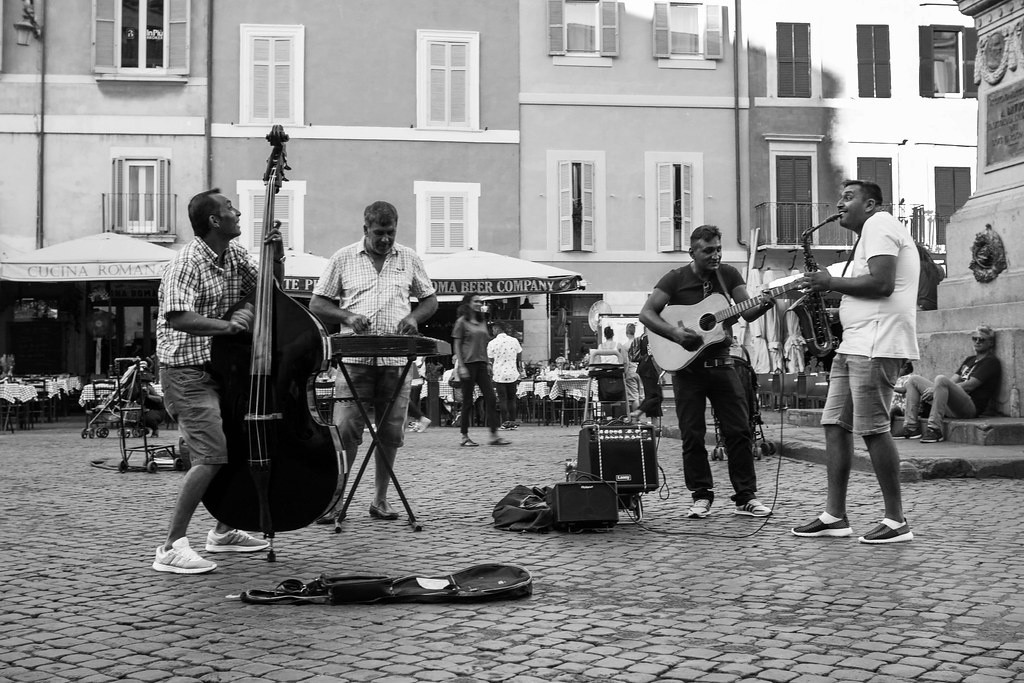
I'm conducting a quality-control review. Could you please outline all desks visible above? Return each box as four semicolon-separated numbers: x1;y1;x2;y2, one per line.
0;376;83;430
421;380;499;424
516;382;534;423
557;378;593;426
535;382;553;426
78;384;164;428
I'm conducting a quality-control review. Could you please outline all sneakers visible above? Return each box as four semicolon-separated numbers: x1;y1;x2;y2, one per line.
791;512;853;537
152;536;217;573
734;500;773;517
892;427;922;439
206;528;270;552
687;499;711;518
919;427;943;442
858;518;913;544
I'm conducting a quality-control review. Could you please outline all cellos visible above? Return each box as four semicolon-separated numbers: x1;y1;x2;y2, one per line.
200;125;349;562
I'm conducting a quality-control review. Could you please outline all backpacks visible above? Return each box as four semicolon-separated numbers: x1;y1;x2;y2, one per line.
628;334;647;362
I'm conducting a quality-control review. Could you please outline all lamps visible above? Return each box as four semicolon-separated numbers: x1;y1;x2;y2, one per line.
517;296;535;309
14;6;41;47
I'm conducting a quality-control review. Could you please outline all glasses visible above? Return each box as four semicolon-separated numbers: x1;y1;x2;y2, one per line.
972;336;984;342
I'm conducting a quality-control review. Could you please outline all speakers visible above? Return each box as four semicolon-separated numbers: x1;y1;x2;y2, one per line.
548;479;620;525
577;425;660;491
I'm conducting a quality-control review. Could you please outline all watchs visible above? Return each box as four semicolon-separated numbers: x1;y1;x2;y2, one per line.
275;256;287;264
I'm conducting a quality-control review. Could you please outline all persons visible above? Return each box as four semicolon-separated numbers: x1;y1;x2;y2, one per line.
451;292;513;445
890;361;913;427
152;188;287;574
409;356;432;433
308;201;438;522
916;245;944;310
545;357;565;371
487;322;522;430
640;224;773;518
892;325;1001;441
597;323;664;432
792;179;921;544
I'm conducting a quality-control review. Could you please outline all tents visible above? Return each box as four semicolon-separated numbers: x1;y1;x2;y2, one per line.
425;247;583;320
248;247;330;299
0;230;177;281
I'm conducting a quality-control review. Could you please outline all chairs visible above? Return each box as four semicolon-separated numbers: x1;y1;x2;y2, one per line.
0;379;18;434
756;372;829;410
315;381;335;425
92;379;120;429
21;378;52;427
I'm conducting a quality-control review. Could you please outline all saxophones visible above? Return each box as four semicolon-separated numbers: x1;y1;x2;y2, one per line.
786;213;843;358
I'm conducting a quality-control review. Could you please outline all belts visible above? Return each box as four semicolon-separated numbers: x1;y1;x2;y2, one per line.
696;359;733;367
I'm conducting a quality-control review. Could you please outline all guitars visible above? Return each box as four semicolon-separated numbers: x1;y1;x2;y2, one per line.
646;277;807;373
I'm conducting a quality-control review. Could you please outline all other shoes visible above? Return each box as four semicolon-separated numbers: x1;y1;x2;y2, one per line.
418;417;432;432
497;422;518;430
316;508;345;524
369;501;398;519
408;422;420;431
488;438;511;445
451;415;461;426
460;440;479;448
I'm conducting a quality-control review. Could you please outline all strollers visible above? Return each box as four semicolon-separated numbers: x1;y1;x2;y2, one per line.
710;344;777;461
80;356;154;439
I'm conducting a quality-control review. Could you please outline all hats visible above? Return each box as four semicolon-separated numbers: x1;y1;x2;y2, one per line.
972;326;995;338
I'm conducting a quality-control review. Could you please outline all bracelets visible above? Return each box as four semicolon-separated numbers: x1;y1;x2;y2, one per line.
488;362;492;364
